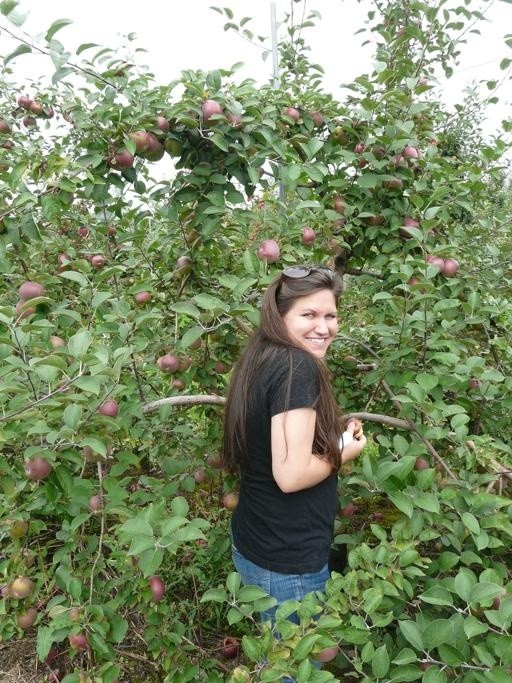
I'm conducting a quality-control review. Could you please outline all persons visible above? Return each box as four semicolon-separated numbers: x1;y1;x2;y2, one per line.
222;264;369;682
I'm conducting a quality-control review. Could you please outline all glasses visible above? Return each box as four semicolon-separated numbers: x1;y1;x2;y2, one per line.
278;265;332;288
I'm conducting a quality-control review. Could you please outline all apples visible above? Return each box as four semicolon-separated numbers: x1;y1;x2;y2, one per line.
1;96;512;682
384;16;407;37
